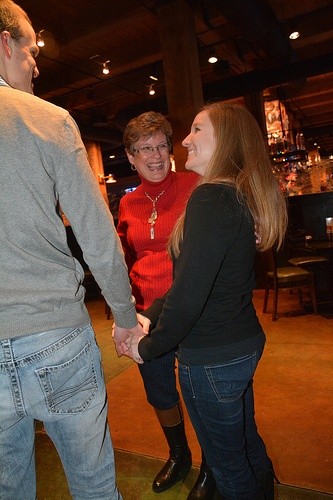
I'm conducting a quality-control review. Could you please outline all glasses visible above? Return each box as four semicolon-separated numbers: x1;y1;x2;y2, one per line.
130;142;172;155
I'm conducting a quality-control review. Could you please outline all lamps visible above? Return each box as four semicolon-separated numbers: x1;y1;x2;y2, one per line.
98;174;117;184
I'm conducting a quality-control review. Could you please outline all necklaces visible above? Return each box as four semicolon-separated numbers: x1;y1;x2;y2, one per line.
142;174;171;240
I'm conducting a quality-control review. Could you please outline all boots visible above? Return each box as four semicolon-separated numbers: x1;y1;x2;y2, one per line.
152;419;192;493
186;439;216;500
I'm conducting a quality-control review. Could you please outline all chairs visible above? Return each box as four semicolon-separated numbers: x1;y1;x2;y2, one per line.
262;246;333;321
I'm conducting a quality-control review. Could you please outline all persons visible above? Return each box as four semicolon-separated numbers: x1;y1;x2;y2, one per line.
0;0;144;500
114;111;218;500
112;100;288;500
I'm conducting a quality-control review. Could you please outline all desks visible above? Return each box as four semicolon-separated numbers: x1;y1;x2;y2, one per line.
287;242;333;311
